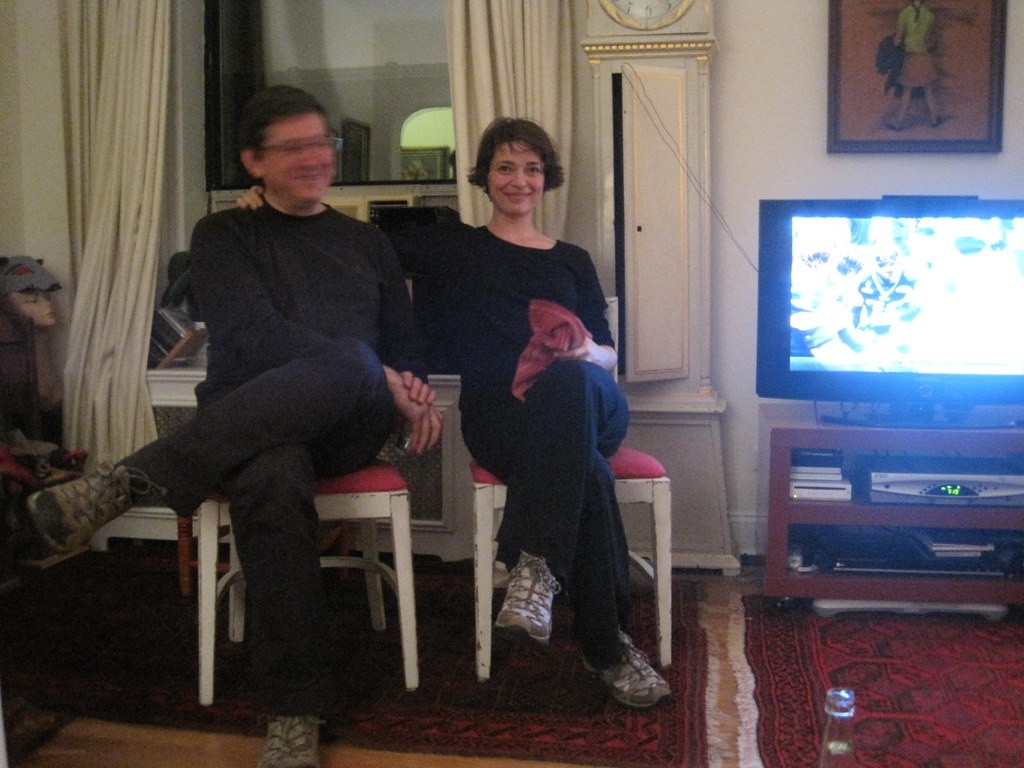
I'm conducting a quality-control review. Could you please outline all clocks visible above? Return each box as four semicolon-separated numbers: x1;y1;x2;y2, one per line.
599;0;693;31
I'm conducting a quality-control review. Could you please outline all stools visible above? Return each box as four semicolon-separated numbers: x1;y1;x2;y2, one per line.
196;459;416;710
471;454;673;682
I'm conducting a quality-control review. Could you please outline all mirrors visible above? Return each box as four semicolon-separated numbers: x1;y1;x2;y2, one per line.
202;0;462;200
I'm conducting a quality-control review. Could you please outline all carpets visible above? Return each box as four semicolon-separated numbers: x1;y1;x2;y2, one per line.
723;585;1024;768
0;541;731;768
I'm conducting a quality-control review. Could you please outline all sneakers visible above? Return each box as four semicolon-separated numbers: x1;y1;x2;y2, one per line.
583;629;670;707
27;460;170;552
493;551;560;648
257;715;326;768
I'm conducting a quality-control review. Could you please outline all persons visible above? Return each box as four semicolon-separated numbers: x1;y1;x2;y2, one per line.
0;254;62;331
236;118;673;710
28;83;443;768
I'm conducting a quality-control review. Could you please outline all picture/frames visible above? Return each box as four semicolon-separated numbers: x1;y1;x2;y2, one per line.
400;146;452;181
340;120;371;183
825;0;1009;152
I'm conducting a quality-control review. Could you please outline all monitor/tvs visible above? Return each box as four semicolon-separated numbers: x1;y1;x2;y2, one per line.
755;196;1023;429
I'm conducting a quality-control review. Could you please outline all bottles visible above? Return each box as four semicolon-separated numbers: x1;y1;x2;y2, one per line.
816;688;858;768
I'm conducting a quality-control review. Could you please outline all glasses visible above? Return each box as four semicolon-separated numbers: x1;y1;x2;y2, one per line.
257;137;344;155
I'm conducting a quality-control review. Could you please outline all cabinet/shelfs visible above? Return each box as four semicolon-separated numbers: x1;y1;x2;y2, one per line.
756;402;1023;605
